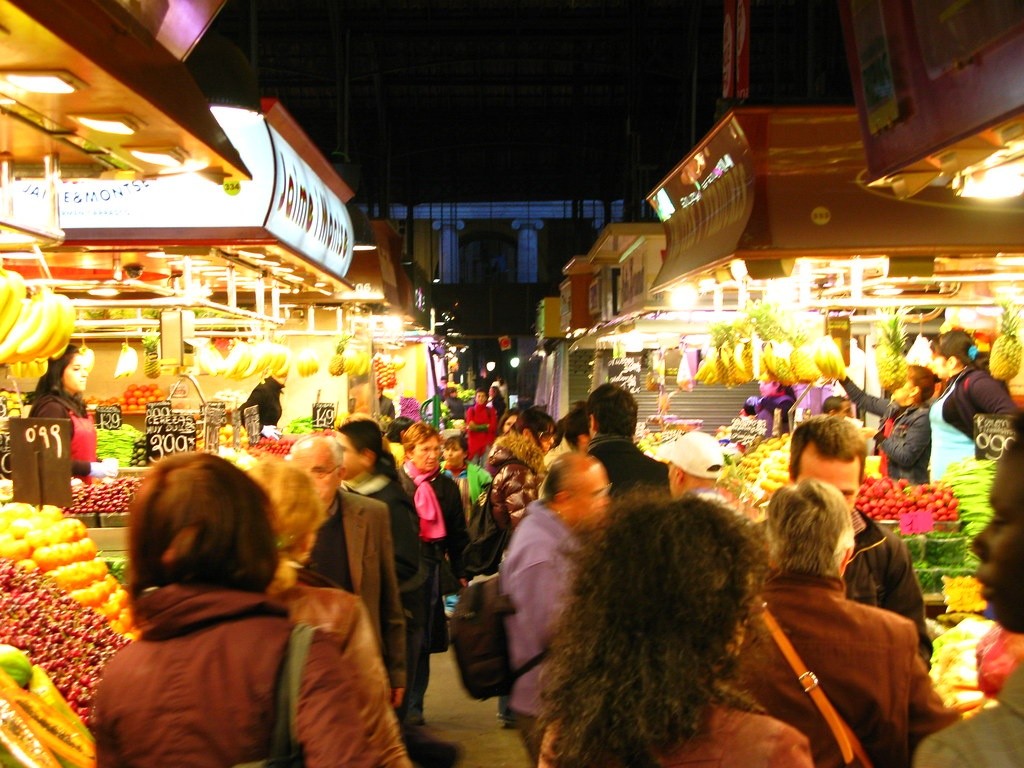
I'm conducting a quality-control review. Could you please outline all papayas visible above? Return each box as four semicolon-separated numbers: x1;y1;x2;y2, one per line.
0;644;98;768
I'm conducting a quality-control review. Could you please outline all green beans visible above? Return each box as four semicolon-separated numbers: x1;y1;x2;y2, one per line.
940;459;995;536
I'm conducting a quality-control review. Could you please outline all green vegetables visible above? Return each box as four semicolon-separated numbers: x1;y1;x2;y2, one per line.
282;415;324;435
895;529;976;593
95;423;142;466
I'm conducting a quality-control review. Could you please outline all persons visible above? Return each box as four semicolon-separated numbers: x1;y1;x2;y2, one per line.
27;345;120;479
223;371;799;768
721;414;960;768
836;364;942;487
93;436;409;768
538;496;812;768
910;414;1024;768
237;369;288;441
927;329;1020;485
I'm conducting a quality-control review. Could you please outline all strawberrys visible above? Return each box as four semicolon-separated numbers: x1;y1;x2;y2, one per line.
854;475;959;522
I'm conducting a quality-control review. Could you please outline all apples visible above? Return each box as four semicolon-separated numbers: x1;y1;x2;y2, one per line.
373;360;396;388
120;384;165;412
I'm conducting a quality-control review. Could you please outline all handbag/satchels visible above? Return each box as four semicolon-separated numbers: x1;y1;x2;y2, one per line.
461;459;538;577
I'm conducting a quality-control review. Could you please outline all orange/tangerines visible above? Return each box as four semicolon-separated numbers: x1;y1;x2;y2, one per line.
0;503;140;642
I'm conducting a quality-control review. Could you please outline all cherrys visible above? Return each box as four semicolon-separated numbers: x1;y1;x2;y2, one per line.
0;556;135;731
61;475;145;512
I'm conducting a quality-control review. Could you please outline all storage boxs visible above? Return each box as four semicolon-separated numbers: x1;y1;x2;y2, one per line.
877;520;900;532
65;513;100;528
97;512;130;527
934;521;962;534
89;527;129;550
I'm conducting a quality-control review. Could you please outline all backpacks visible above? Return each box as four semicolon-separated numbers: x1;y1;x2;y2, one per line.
449;575;557;699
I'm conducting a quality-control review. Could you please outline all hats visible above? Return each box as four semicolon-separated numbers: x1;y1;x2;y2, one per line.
656;431;724;480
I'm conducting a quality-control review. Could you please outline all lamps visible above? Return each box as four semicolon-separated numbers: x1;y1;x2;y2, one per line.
122;145;190;168
270;264;295;273
283;268;306;281
234;247;266;258
66;111;149;136
256;255;282;267
302;273;316;288
314;283;334;297
884;170;942;200
0;68;91;96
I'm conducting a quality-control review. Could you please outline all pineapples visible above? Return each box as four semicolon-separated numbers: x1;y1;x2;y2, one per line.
327;338;348;376
712;302;822;386
645;365;659;390
989;292;1024;382
875;304;913;390
142;333;162;379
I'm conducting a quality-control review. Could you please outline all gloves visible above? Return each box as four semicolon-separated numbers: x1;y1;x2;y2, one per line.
260;425;283;440
88;462;118;477
101;457;119;476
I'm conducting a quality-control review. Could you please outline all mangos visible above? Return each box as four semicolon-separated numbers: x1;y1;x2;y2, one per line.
741;432;790;493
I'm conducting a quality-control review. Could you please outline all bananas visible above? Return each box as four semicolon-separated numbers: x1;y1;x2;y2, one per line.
114;343;138;379
0;262;75;379
343;353;373;375
80;346;98;371
814;334;846;381
694;365;718;385
393;355;405;371
734;343;745;372
721;347;729;367
197;347;319;378
765;340;779;374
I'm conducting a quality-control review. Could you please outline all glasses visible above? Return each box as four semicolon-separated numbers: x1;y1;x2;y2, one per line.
593;482;613;500
537;431;557;442
313;464;341;480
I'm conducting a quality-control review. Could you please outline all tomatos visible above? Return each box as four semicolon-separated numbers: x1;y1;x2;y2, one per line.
249;436;294;458
